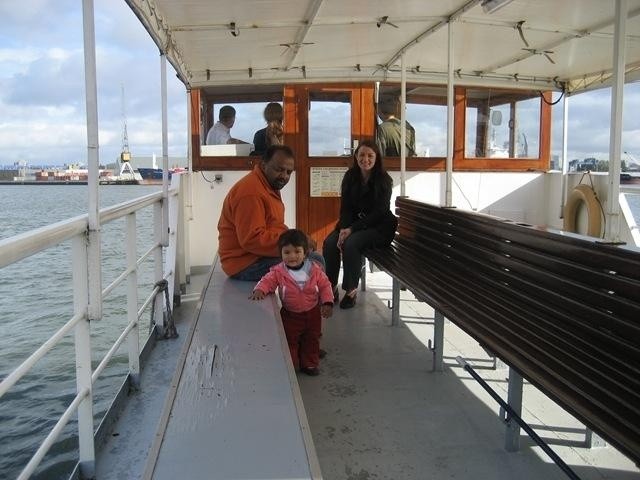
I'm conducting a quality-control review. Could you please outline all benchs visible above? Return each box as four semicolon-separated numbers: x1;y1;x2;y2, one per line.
361;196;640;480
141;255;323;480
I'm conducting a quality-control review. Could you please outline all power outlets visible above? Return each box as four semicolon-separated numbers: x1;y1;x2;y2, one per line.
215;175;223;183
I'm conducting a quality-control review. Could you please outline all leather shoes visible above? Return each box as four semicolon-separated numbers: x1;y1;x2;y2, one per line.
333;288;339;302
339;292;358;309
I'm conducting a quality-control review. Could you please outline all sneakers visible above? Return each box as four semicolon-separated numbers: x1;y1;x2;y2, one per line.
300;366;319;376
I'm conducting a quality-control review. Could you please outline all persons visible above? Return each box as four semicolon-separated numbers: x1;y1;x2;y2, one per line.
248;229;335;376
205;106;252;144
253;102;283;150
322;141;399;309
376;99;417;158
216;144;328;359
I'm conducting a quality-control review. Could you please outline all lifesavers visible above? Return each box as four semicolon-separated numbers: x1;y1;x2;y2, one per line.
563;184;601;238
